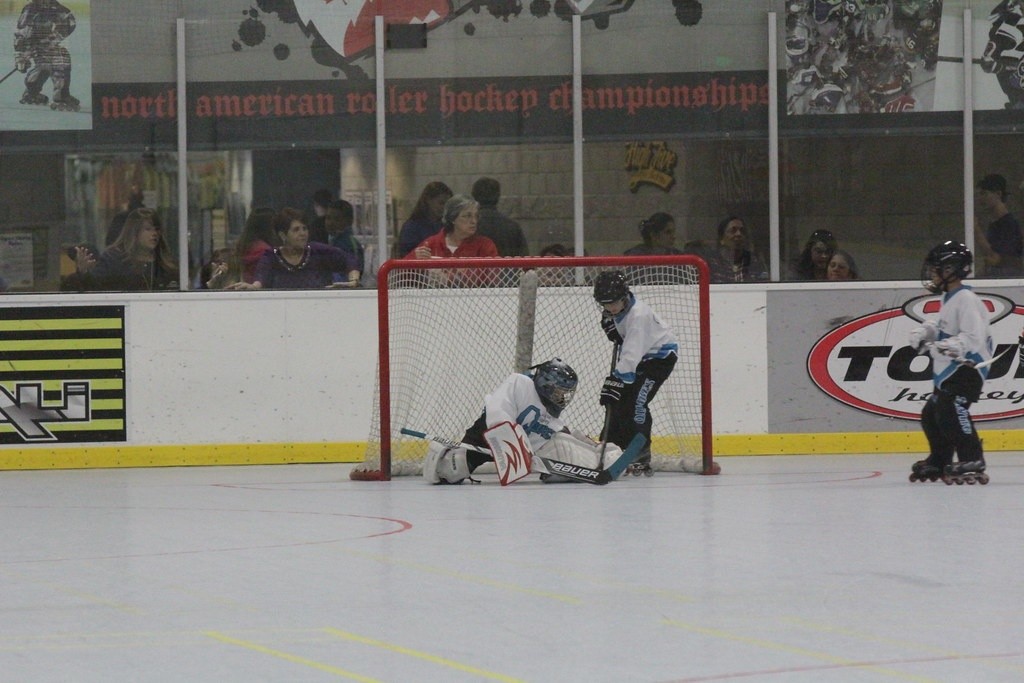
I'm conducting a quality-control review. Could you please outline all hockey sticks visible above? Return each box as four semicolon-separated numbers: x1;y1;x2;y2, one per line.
399;427;647;486
920;339;1013;370
540;340;619;483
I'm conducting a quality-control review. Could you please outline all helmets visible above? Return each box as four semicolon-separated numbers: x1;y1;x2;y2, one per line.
925;241;974;278
594;270;629;303
534;358;578;413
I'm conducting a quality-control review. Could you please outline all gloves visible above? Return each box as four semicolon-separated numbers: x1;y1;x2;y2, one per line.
930;335;966;362
909;319;939;353
600;377;624;408
600;318;623;345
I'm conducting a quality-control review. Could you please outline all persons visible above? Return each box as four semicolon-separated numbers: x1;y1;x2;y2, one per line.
534;244;593;288
909;239;993;485
468;177;531;288
106;184;147;248
13;0;81;112
592;269;678;477
391;182;457;288
974;173;1024;278
74;207;180;290
200;189;364;290
422;357;627;486
400;193;500;288
621;211;857;285
60;241;100;293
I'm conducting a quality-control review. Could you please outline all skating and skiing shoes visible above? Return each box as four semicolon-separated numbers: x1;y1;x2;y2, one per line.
623;448;655;477
909;455;951;482
943;457;989;485
